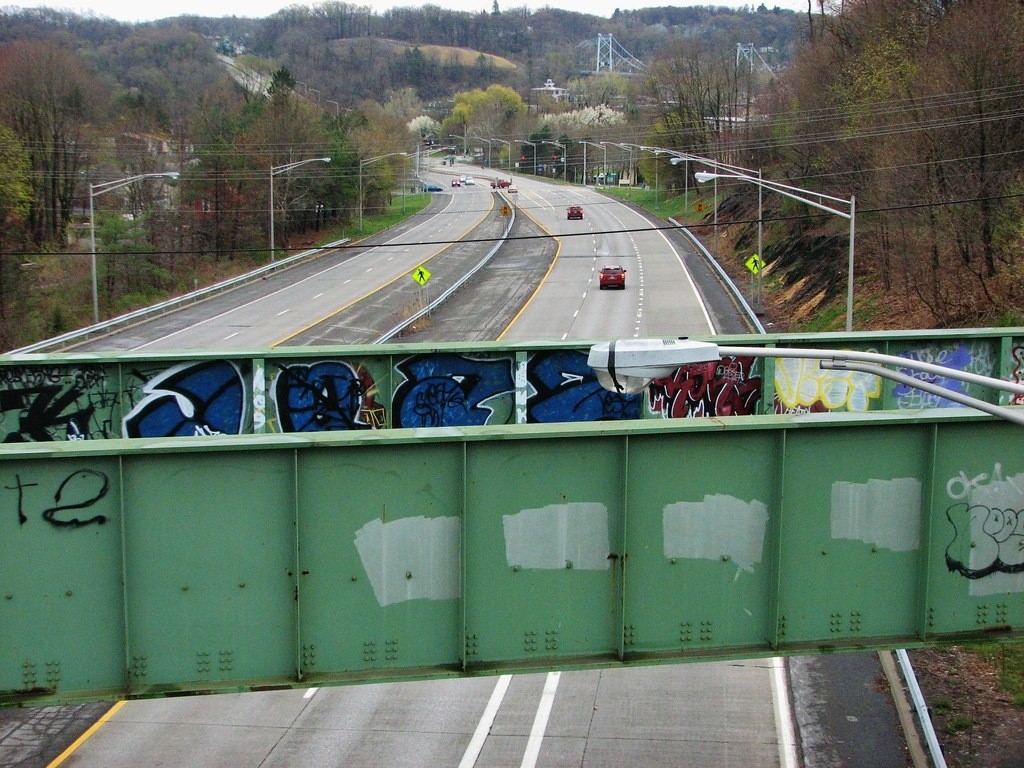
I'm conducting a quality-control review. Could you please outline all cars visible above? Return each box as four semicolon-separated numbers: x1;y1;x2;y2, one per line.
565;203;585;222
598;265;626;290
465;177;475;185
451;177;460;187
508;185;518;193
460;175;466;182
421;184;444;192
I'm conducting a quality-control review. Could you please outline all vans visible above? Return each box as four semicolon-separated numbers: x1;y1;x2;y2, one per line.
491;176;512;189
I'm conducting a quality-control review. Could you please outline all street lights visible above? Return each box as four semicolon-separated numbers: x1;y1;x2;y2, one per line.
620;140;855;335
269;157;331;263
541;140;568;182
411;137;451;182
473;136;491;169
579;141;607;190
449;135;467;158
357;152;407;233
514;140;538;177
601;141;632;198
491;138;510;174
89;169;178;326
